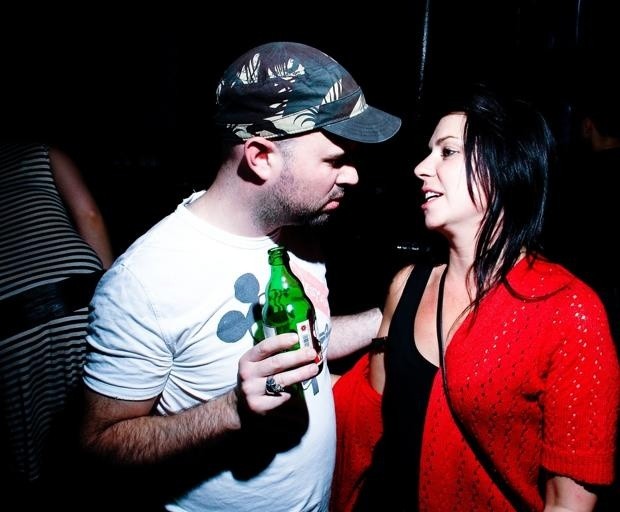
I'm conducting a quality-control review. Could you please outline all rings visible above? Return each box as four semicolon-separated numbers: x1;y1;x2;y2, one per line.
266;376;285;396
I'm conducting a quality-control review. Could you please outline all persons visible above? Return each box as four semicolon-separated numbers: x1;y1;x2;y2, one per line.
0;137;118;511
82;39;386;512
328;86;619;512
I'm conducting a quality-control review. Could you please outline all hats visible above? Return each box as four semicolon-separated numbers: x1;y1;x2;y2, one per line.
210;38;405;145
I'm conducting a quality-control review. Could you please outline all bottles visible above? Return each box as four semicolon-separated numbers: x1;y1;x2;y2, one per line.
261;246;325;385
